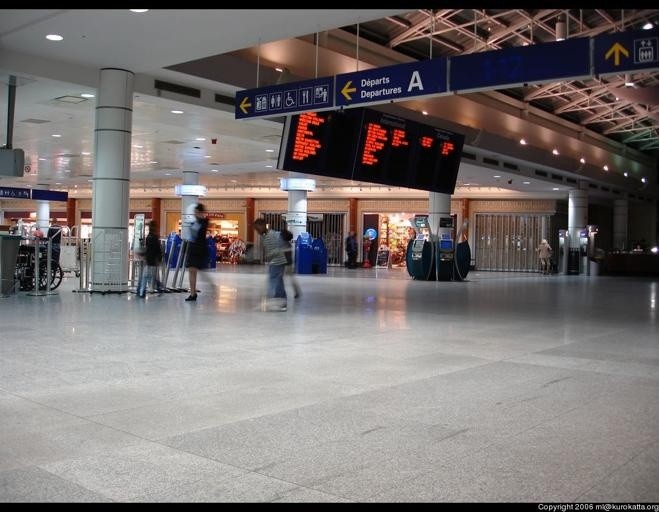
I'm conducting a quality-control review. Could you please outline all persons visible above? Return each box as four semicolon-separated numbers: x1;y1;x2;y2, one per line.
345;230;355;269
351;233;359;267
47;216;61;247
251;217;289;313
144;220;163;296
363;235;372;268
278;219;302;300
534;238;553;275
28;223;43;246
183;203;212;303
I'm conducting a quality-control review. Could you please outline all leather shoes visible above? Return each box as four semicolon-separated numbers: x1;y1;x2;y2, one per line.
185;293;197;301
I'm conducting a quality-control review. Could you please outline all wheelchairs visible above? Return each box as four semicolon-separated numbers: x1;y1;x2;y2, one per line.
14;233;63;293
